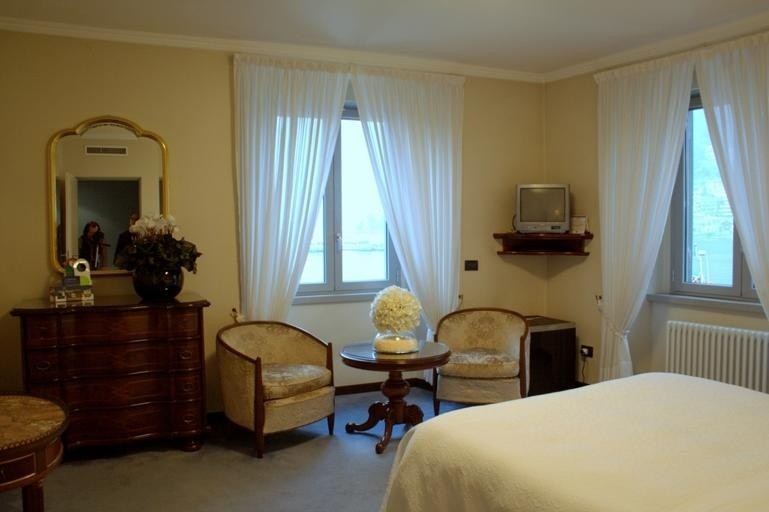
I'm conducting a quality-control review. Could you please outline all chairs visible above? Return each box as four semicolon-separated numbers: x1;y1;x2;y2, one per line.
216;320;337;460
433;307;530;415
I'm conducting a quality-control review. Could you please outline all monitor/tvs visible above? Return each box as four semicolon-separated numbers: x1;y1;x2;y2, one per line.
515;183;570;234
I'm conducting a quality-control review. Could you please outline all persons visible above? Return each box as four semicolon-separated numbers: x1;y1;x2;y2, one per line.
113;213;140;269
78;221;107;271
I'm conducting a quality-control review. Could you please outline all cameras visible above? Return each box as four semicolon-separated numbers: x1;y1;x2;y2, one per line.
91;232;106;244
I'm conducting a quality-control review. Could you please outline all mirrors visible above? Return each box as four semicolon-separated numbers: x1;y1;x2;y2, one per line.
45;115;171;280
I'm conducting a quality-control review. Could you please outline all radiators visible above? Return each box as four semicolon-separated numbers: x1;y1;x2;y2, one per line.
665;320;769;394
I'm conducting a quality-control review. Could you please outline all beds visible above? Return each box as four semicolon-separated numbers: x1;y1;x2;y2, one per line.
387;371;768;512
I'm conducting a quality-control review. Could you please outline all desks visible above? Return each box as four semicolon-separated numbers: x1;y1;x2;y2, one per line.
0;392;66;512
342;341;451;453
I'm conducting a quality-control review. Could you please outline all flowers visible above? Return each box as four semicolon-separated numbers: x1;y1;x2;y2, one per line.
368;285;423;335
117;214;201;275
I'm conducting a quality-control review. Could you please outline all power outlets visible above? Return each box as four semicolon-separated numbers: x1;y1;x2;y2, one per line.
581;344;593;359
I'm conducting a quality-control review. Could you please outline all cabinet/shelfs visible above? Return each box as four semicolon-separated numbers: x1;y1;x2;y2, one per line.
492;231;594;256
10;290;211;464
530;314;577;396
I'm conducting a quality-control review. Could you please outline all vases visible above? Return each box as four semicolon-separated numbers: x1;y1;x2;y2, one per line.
375;333;422;353
131;260;183;304
